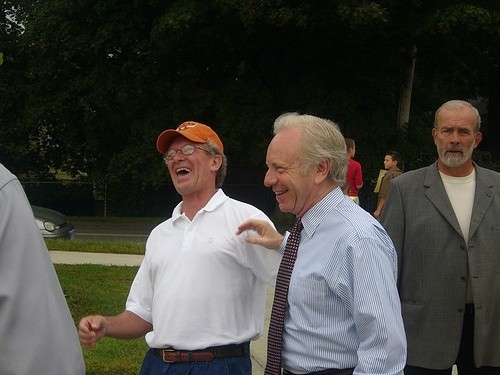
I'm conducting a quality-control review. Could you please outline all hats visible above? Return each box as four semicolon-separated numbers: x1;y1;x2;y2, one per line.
157;121;223;154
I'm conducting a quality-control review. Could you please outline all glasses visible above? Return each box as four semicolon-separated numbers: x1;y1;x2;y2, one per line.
164;144;212;160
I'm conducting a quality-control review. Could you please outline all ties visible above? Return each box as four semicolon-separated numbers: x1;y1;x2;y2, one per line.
263;217;305;375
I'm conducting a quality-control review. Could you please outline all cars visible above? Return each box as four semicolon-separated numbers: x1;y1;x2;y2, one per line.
29;205;75;240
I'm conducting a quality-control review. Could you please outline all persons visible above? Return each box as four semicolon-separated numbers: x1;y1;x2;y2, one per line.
380;100;500;375
236;113;406;375
345;138;364;206
78;121;283;375
0;162;87;375
374;151;404;219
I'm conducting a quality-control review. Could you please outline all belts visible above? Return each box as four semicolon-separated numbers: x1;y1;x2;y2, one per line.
157;340;250;363
